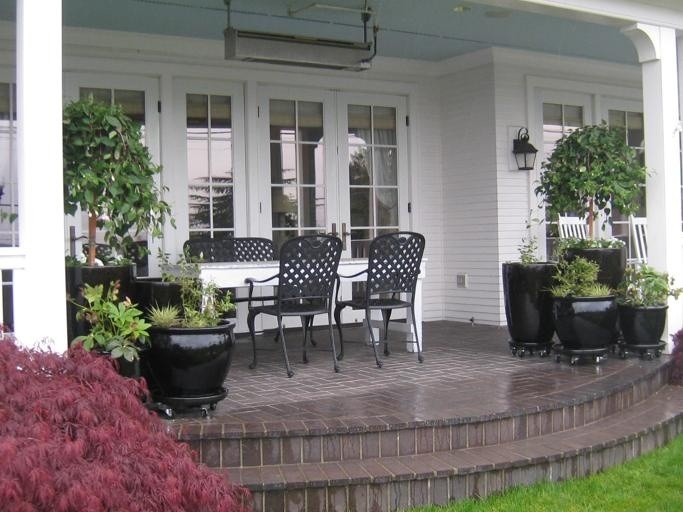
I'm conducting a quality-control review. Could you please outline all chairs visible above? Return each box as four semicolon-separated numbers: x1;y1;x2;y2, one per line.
181;231;424;375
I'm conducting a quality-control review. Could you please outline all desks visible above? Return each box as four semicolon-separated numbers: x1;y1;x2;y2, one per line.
160;257;428;354
